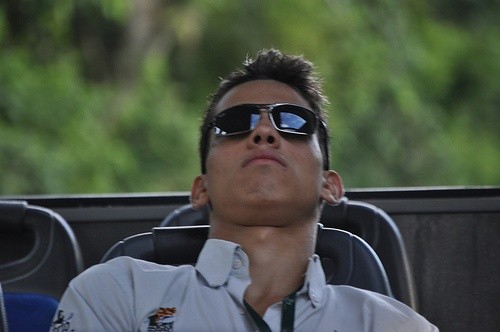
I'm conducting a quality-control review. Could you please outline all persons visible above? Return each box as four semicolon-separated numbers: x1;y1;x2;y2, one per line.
48;48;441;331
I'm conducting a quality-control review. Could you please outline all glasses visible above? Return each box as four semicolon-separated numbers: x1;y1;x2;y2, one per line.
203;102;328;137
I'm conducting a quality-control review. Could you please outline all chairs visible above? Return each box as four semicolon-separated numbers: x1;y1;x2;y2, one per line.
100;223;393;297
0;200;84;301
160;196;419;312
